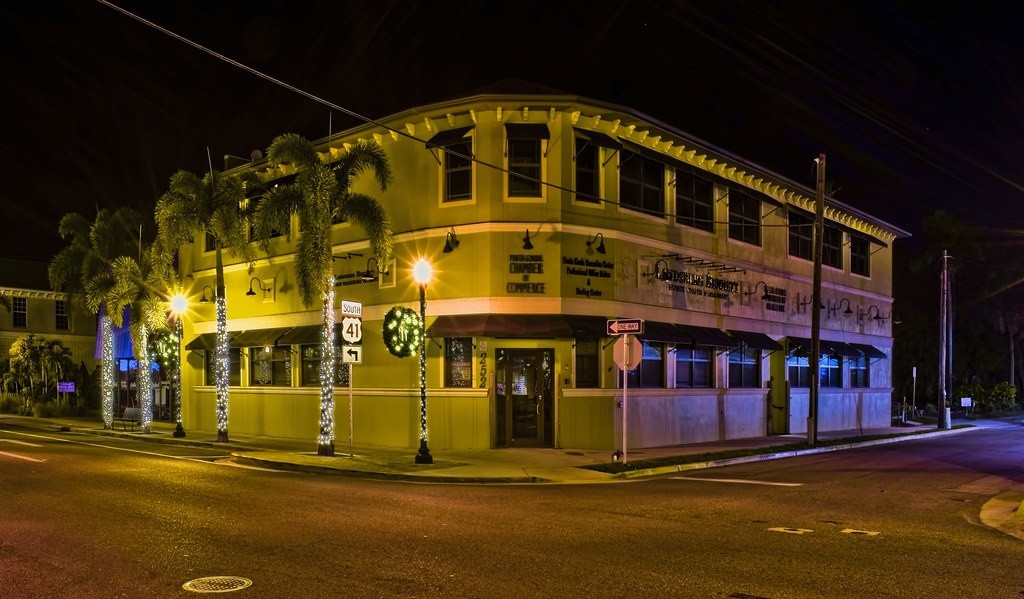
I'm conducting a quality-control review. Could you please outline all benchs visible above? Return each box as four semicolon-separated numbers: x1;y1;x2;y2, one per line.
112;407;143;432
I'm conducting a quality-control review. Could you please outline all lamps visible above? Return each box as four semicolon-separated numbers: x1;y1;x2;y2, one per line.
743;281;771;300
245;277;271;296
800;293;826;310
860;304;882;320
443;231;460;253
332;252;363;262
831;298;853;313
661;252;747;275
882;310;902;325
522;229;534;250
199;285;216;304
361;257;390;281
587;232;606;255
642;259;671;282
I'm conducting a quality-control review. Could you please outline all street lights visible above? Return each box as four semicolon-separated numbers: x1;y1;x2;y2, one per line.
170;295;187;437
411;258;433;464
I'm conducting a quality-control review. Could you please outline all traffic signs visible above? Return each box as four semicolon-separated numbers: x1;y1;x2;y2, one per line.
607;318;642;335
